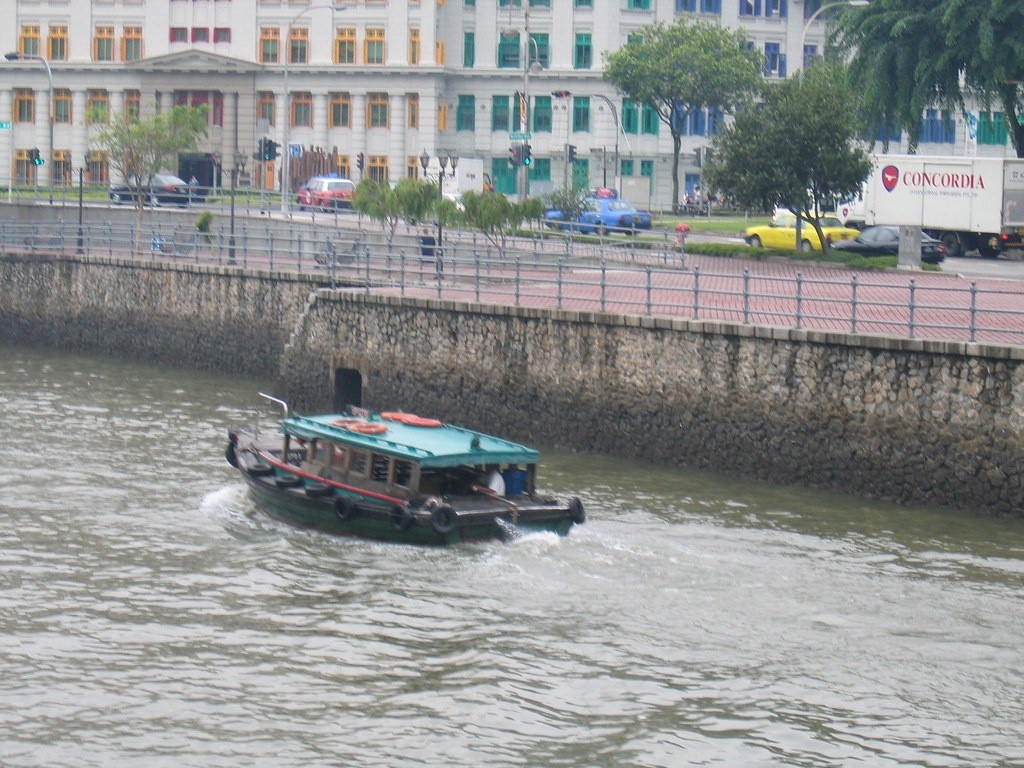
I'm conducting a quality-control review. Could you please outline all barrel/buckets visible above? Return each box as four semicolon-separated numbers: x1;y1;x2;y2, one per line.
502;468;527;496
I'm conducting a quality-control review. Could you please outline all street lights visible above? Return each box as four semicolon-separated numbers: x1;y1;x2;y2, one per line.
519;36;543;209
4;52;56;206
214;149;250;265
419;147;459;280
798;1;871;93
63;150;92;254
279;4;346;214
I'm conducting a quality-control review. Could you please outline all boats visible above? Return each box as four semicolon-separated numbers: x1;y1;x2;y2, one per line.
223;393;587;547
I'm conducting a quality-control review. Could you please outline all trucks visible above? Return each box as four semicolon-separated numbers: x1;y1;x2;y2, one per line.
835;152;1024;259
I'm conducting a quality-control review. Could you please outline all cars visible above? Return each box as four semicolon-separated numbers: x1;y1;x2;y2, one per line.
742;211;861;257
109;171;190;208
298;174;358;213
543;194;652;237
828;225;945;264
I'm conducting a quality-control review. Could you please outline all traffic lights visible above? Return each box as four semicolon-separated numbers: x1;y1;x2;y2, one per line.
25;148;44;167
551;91;571;97
523;145;531;165
356;153;363;170
258;136;277;159
508;147;520;166
569;144;576;163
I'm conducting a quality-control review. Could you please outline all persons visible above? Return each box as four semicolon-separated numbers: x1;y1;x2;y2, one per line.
473;464;524;499
681;184;708;215
417;230;435;261
188;176;200;195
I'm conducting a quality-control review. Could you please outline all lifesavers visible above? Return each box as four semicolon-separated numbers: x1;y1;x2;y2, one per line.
351;422;387;434
380;411;418;422
274;475;303;487
305;484;334;497
389;505;411;533
402;417;442;427
430;506;459;535
333;496;354;521
570;496;585;524
248;463;274;476
333;419;365;428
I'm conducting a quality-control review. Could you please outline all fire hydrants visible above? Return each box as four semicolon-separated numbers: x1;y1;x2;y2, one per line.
675;225;692;248
